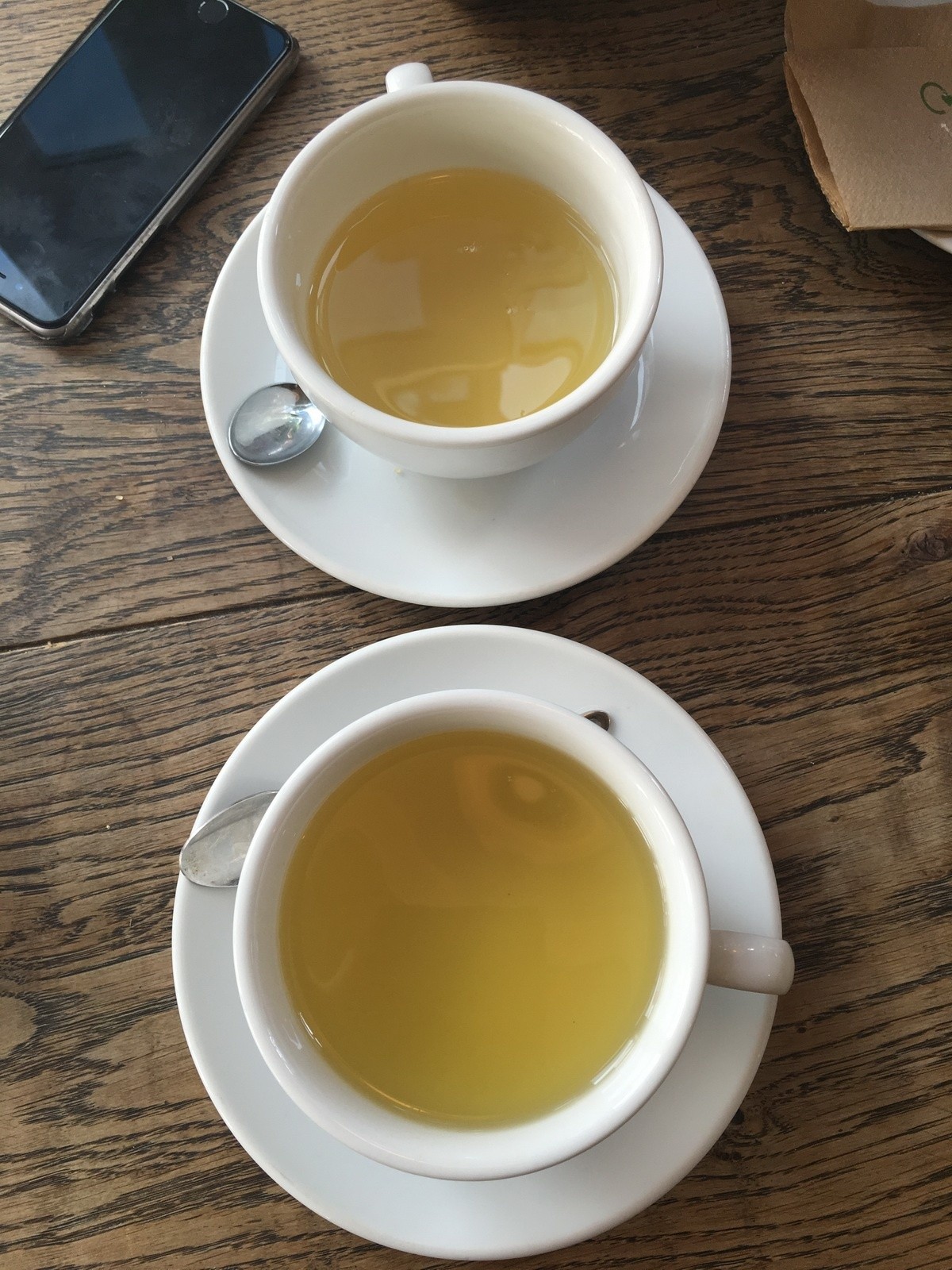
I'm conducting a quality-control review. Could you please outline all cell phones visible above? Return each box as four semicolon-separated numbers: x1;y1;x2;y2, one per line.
1;1;297;343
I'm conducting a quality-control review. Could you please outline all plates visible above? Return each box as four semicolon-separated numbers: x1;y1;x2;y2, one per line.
169;623;786;1261
200;175;730;609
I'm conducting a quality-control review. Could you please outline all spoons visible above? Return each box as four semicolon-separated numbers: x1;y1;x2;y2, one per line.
176;709;610;889
229;381;326;467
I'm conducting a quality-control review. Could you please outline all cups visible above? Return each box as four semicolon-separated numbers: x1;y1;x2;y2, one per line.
232;689;795;1183
257;57;663;478
